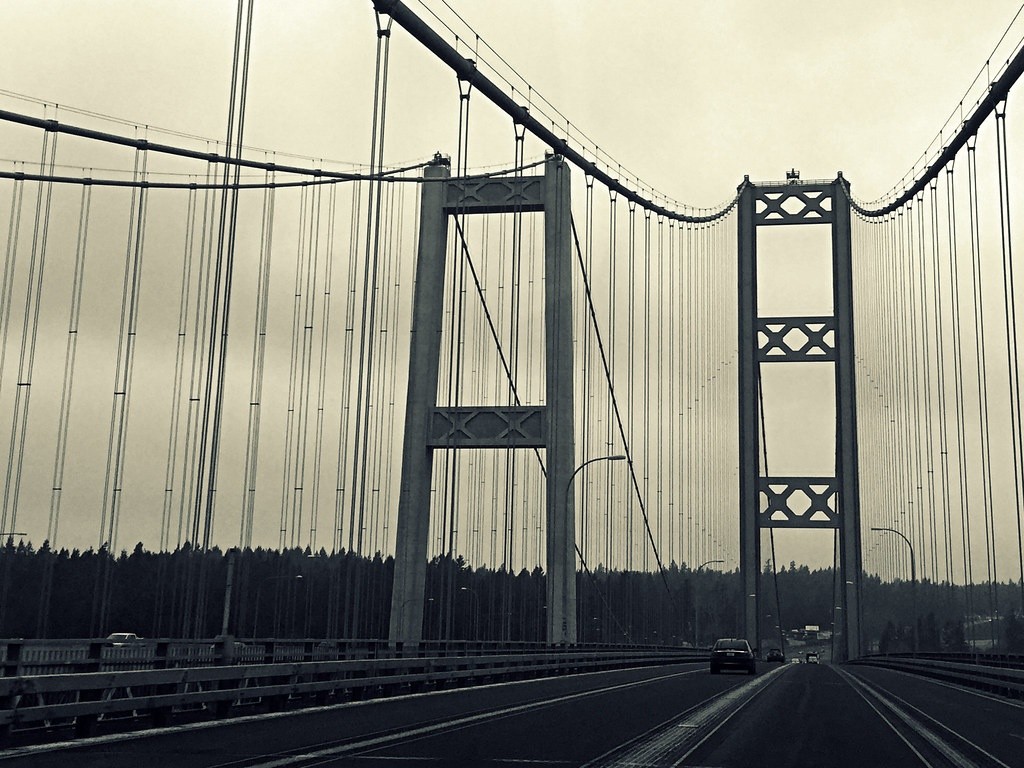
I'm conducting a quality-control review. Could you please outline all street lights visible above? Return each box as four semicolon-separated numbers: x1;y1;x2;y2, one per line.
871;527;919;650
558;455;627;671
461;587;476;649
693;560;724;647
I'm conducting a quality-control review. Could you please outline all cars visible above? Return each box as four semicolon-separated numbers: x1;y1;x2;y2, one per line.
106;633;146;647
791;647;826;665
709;638;757;675
766;648;786;663
